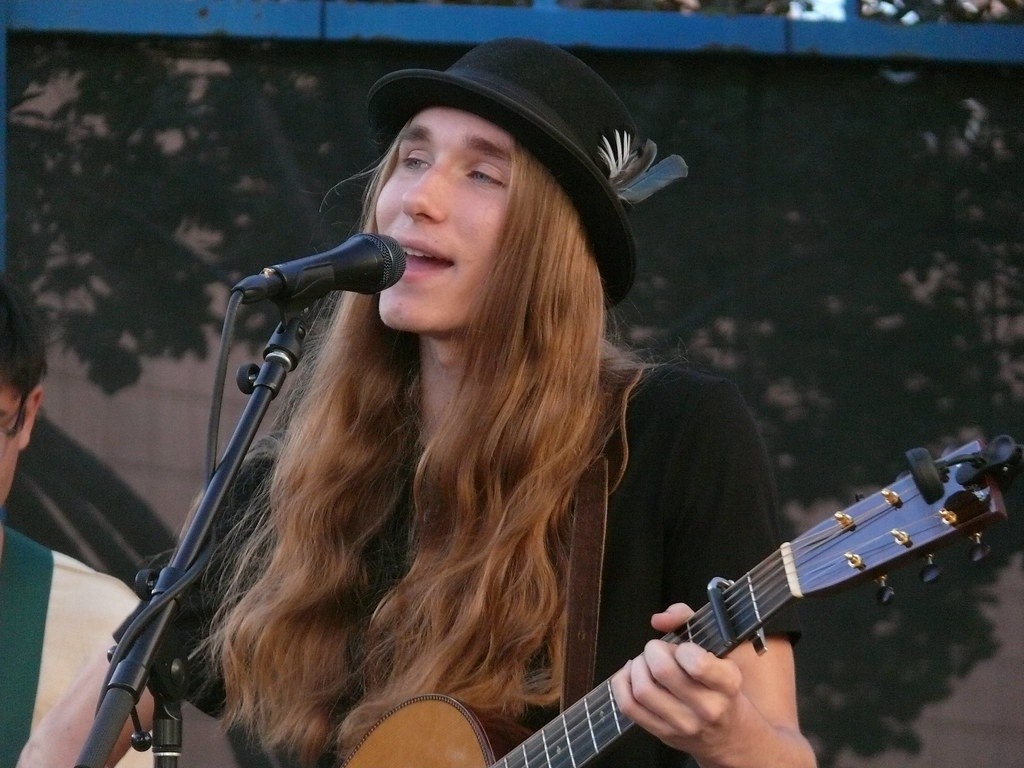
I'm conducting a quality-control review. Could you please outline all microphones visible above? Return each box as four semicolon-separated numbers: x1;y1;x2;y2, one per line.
230;233;406;304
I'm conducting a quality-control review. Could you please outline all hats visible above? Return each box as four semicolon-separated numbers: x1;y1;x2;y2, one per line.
366;38;689;311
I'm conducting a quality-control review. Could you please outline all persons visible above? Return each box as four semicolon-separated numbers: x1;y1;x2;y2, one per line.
0;276;156;768
12;37;818;768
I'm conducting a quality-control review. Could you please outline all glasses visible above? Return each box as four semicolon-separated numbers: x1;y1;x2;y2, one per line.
0;391;28;459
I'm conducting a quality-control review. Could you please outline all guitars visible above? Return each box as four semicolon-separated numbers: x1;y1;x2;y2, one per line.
339;433;1024;768
